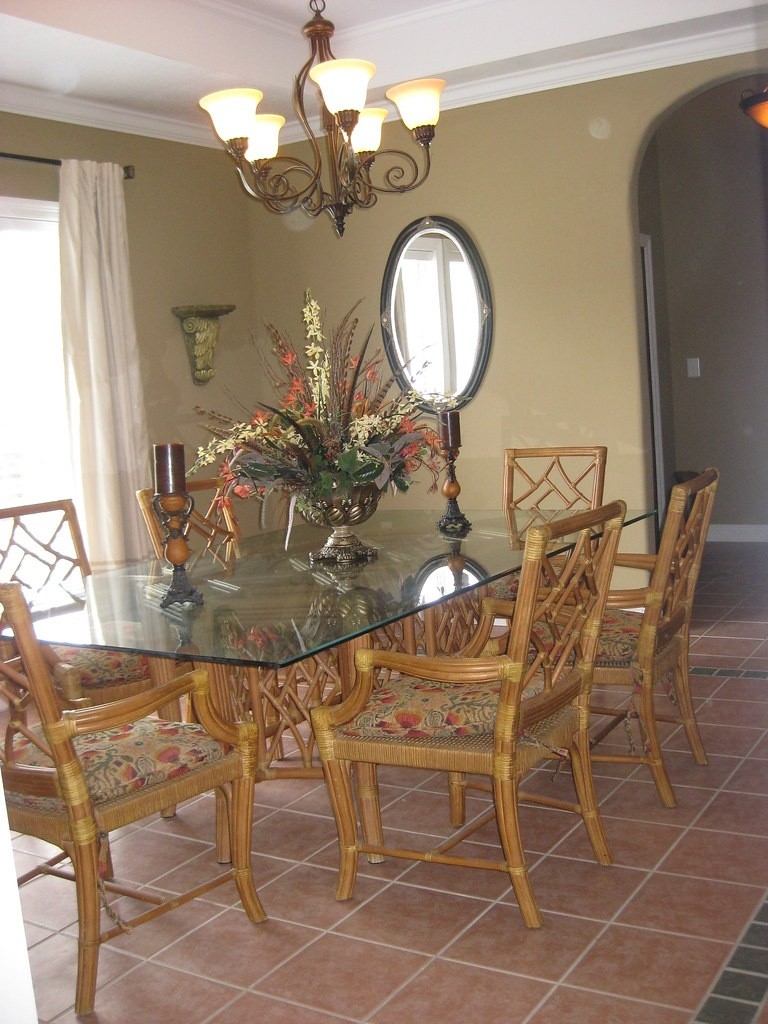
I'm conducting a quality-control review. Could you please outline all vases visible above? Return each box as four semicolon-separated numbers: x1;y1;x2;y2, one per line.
284;476;381;567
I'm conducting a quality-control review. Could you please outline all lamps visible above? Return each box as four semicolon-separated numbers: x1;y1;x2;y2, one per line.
197;0;447;238
739;85;768;129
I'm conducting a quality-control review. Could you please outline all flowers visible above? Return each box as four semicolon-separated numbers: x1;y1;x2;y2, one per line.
177;286;470;497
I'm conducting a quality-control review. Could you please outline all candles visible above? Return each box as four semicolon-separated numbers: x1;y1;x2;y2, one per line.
154;442;187;494
438;411;460;447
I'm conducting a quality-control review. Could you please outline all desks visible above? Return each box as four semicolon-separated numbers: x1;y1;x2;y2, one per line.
15;505;661;874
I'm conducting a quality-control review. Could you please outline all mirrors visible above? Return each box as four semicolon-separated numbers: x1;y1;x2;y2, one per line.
376;215;493;412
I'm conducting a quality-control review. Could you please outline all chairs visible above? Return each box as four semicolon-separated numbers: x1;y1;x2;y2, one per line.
497;466;723;806
1;588;271;1016
136;476;285;764
486;447;607;604
310;498;625;929
0;497;201;728
